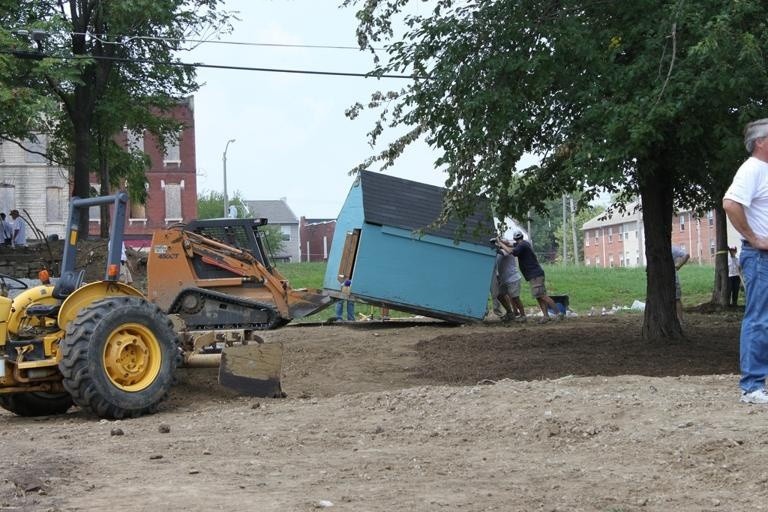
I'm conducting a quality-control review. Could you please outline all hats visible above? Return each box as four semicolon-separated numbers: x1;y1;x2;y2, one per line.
513;231;523;240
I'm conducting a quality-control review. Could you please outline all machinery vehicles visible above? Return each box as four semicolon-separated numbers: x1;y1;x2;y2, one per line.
0;183;297;418
146;217;339;334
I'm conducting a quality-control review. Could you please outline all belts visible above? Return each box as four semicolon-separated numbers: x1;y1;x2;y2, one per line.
744;241;752;247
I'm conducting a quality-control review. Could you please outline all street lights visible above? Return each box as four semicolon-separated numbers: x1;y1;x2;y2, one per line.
221;137;238;217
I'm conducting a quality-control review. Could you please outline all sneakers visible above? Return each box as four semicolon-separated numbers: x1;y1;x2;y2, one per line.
514;315;526;322
739;387;768;404
493;308;502;316
538;316;550;324
500;312;515;321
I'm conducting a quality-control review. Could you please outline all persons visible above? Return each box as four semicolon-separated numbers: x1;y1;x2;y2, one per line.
9;210;26;249
485;270;503;317
722;118;768;407
496;230;561;325
727;246;740;307
670;244;689;325
108;239;135;286
496;238;527;323
0;212;12;248
335;299;356;322
382;307;389;320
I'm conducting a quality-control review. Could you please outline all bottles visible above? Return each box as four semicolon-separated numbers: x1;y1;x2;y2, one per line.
564;304;623;318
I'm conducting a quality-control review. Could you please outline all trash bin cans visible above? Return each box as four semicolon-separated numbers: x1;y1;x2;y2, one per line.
547;295;569;316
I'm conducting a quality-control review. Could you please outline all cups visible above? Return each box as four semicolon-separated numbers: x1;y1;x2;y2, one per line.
227;206;237;219
